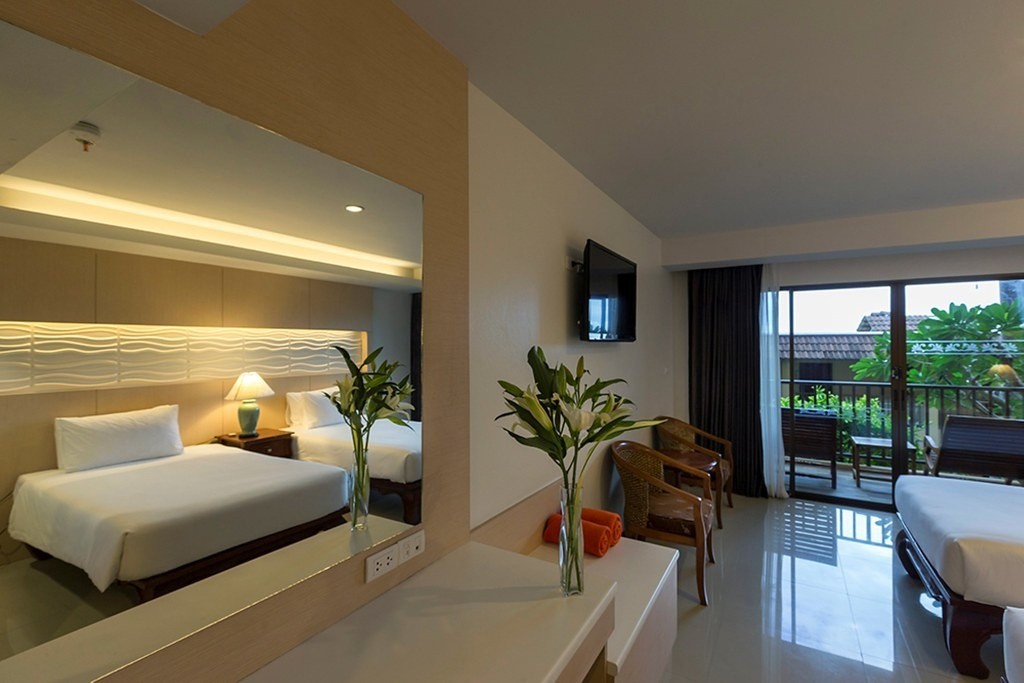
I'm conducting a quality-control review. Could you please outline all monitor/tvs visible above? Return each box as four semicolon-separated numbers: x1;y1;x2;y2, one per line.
579;239;637;342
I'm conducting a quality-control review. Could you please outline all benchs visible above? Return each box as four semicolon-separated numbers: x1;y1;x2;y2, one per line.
922;414;1024;486
768;497;838;567
780;405;837;488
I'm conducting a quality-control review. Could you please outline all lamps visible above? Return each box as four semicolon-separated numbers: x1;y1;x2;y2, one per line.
223;369;277;438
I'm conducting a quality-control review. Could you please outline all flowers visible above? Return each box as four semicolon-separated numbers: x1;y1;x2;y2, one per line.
494;345;668;484
319;345;415;450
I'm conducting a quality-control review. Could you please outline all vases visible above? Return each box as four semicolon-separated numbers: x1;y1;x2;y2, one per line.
350;450;369;530
560;483;585;596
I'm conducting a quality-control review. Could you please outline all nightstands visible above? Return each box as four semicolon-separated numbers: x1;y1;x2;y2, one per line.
214;428;295;459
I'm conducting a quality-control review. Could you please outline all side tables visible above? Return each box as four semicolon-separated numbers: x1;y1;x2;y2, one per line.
657;448;718;497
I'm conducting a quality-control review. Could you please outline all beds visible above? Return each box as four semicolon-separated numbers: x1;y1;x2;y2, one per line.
9;443;350;601
894;474;1024;678
1003;605;1024;683
277;414;421;524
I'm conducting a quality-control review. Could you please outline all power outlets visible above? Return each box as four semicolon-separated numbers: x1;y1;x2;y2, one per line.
566;256;583;272
366;543;399;582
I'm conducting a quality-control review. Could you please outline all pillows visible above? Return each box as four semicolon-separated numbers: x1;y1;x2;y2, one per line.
54;402;184;472
287;386;350;428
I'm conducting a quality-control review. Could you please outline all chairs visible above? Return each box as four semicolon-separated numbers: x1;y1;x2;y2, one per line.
654;415;734;528
611;438;716;607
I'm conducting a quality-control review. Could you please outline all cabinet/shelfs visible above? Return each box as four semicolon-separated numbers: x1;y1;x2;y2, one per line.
241;542;616;683
528;534;680;682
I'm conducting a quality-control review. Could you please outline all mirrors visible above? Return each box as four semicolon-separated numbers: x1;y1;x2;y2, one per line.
0;19;423;660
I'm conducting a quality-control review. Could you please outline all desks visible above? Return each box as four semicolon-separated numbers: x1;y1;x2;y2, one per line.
851;436;916;488
